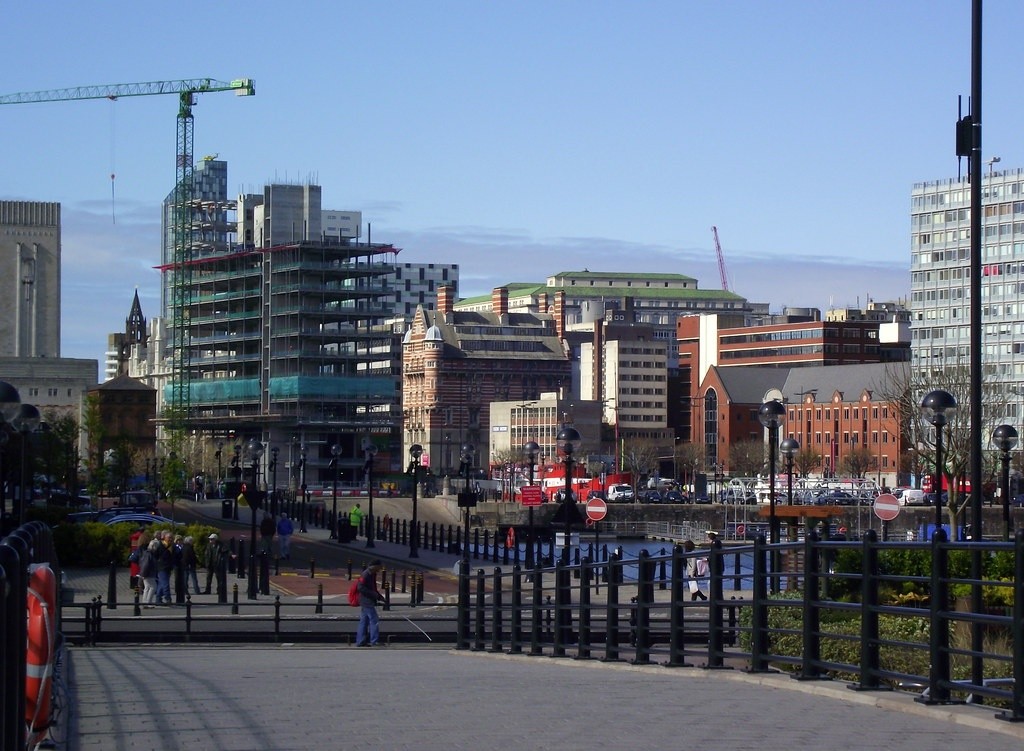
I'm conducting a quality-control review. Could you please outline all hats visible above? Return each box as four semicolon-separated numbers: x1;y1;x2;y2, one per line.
208;534;217;540
369;559;383;565
174;535;183;544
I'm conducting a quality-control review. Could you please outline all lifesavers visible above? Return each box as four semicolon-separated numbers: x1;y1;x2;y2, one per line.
383;514;390;530
242;484;246;492
507;527;514;549
738;526;746;534
840;527;846;534
586;518;593;525
25;567;56;744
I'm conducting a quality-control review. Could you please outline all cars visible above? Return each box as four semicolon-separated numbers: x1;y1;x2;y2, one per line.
541;491;548;503
1010;494;1024;508
716;485;967;507
586;482;712;505
555;487;578;504
13;474;186;532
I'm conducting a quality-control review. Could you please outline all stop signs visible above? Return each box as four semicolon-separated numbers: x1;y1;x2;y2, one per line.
585;498;607;521
873;493;901;521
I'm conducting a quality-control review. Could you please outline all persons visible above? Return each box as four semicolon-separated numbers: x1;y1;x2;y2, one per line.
350;503;364;541
277;513;294;560
707;533;724;600
202;534;229;594
127;530;203;609
683;541;707;601
356;559;385;647
260;512;276;558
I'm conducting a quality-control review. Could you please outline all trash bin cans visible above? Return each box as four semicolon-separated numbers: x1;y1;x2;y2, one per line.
338;517;351;543
497;490;502;500
222;500;232;519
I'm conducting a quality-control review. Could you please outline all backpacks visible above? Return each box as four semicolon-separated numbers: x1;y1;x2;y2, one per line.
347;576;367;607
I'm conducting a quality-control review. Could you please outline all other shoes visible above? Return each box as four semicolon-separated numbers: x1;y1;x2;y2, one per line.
202;591;210;594
359;643;370;647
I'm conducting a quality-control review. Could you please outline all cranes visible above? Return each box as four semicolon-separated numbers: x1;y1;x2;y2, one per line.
1;76;259;490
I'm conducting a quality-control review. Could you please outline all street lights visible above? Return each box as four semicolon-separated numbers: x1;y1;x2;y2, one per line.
922;390;958;528
365;445;379;548
330;443;342;539
270;446;280;520
245;441;264;600
523;440;540;583
779;438;800;538
409;444;423;558
460;443;475;574
992;424;1019;542
758;400;788;597
217;441;224;479
555;427;582;644
299;445;308;534
233;444;242;521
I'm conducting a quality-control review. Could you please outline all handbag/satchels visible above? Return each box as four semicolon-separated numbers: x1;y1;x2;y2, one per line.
128;549;139;563
